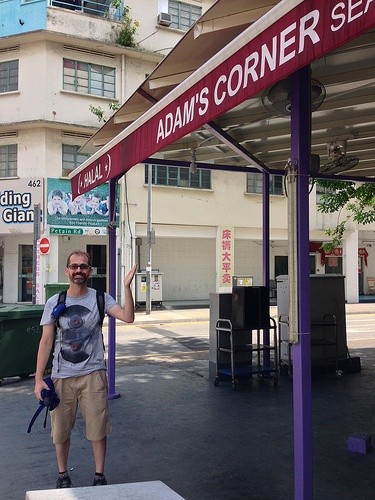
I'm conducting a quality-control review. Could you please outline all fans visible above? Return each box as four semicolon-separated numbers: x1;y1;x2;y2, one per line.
261;76;327;118
320;142;361;174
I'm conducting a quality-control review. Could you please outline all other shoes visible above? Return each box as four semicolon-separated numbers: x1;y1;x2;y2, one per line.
93;477;108;485
56;476;73;488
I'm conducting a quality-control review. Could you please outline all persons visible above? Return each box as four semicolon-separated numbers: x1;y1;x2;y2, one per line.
47;190;120;217
34;252;138;488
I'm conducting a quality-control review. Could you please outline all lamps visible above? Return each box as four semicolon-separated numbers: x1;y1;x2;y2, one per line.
189;149;197;173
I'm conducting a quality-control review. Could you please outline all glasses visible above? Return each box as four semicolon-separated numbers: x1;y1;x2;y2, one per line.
68;264;89;270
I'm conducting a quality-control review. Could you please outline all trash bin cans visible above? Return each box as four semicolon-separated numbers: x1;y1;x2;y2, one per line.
0;303;55;384
44;284;70;304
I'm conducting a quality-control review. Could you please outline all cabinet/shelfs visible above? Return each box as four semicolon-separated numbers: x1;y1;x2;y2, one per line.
278;312;338;382
213;317;278;391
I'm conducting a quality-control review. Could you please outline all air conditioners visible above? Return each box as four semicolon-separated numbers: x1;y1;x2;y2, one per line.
157;12;172;26
